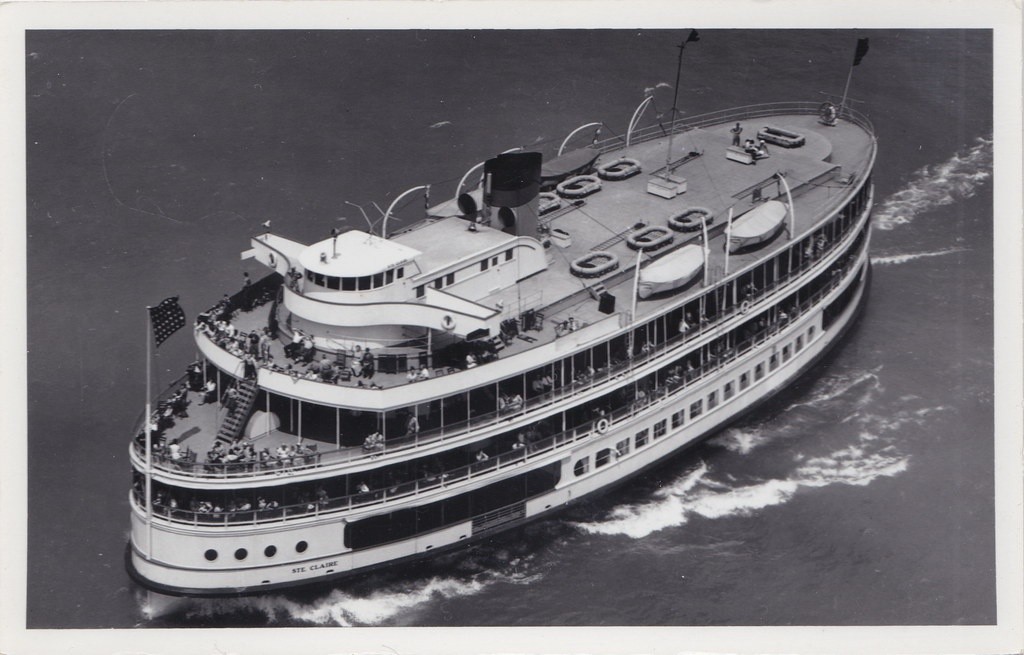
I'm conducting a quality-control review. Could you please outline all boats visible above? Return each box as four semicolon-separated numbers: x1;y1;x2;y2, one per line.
121;33;880;619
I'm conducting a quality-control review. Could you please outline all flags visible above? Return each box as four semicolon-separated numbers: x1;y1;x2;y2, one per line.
149;294;187;346
853;38;870;65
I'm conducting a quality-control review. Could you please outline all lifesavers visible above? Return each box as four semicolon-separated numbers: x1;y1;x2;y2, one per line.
741;300;749;314
597;419;608;433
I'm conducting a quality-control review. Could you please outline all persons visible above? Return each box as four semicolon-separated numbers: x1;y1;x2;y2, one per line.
554;316;577;337
196;271;385;392
357;372;553;499
730;119;769;160
465;351;478;369
137;378;331;522
405;364;430;383
803;235;843;290
576;301;791;428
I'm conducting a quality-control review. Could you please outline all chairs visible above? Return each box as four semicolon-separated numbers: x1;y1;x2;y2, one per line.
137;298;588;475
